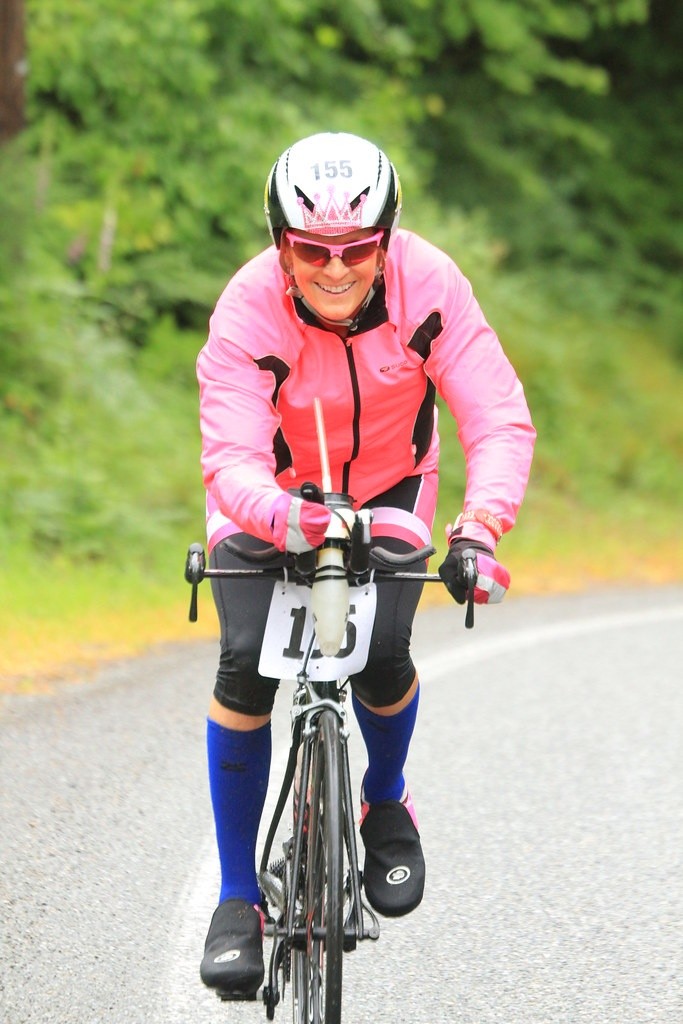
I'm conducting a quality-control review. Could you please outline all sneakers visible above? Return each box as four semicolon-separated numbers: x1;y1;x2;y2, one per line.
359;768;425;916
199;886;266;988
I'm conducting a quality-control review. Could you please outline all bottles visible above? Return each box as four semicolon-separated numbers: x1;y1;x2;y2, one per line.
292;739;320;835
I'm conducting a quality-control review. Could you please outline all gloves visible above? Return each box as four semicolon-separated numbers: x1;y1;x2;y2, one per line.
269;492;330;554
438;537;513;605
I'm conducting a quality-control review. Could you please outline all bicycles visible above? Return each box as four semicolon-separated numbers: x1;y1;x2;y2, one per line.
188;480;481;1024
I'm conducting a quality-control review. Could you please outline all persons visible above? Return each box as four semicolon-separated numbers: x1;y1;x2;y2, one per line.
194;130;537;993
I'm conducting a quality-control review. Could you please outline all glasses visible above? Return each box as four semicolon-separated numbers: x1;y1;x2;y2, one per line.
284;230;384;265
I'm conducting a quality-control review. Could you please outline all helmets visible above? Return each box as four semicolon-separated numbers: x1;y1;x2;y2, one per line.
264;132;401;251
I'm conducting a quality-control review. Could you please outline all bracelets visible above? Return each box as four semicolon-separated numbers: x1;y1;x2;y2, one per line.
456;509;504;545
